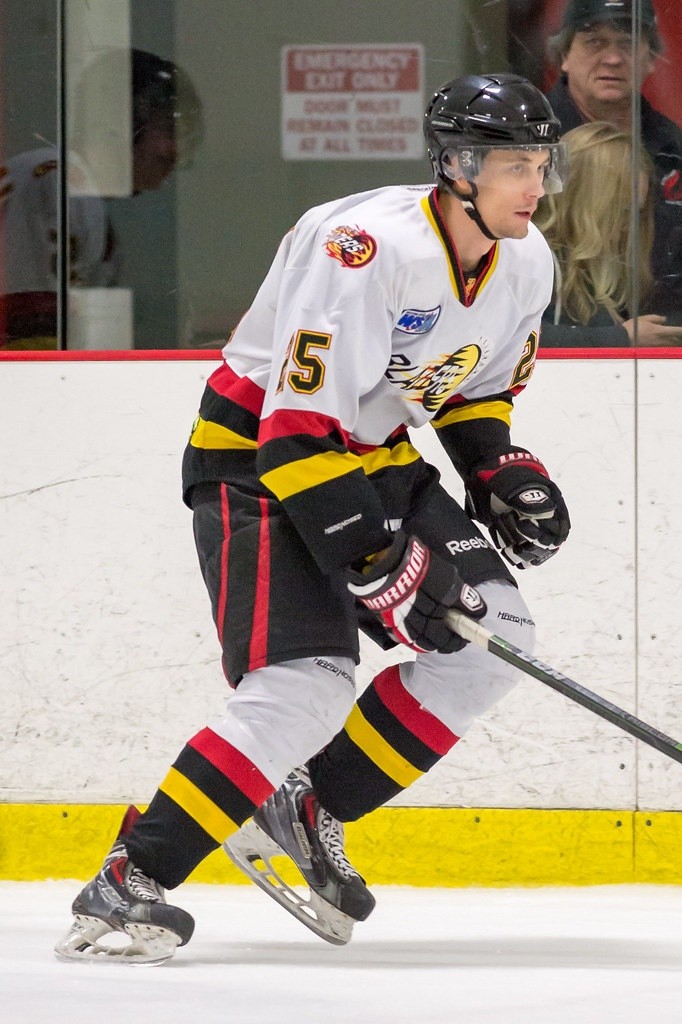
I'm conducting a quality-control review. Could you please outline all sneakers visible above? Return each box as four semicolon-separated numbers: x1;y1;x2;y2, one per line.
223;762;375;946
54;805;195;966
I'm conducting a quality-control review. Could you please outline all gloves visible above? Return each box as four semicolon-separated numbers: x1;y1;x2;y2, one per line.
464;445;571;570
344;529;489;656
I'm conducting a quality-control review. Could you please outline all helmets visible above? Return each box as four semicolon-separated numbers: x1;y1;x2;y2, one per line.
72;48;206;146
423;71;565;192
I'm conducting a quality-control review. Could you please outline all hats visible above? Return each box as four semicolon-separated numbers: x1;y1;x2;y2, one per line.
562;0;656;35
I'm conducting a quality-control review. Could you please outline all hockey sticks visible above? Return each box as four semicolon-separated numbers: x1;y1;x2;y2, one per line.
447;603;682;769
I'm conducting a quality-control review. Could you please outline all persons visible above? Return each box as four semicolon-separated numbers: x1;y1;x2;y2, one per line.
1;50;205;348
545;1;681;312
65;69;570;964
533;122;679;351
